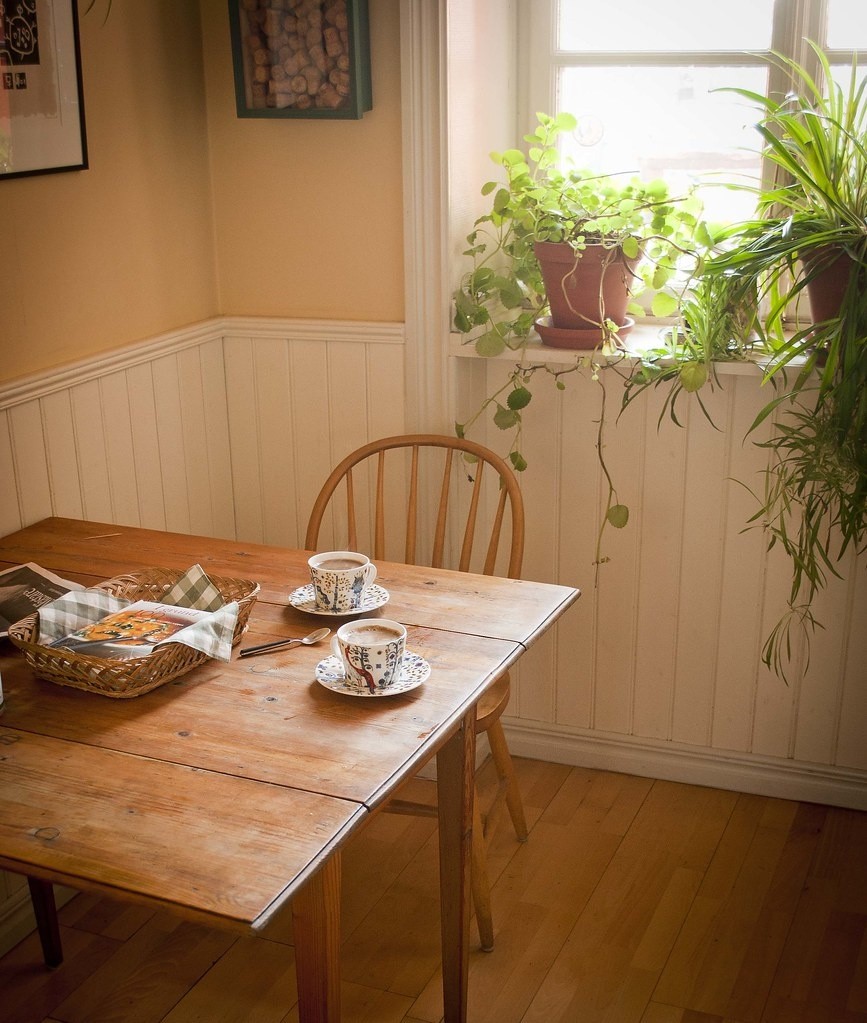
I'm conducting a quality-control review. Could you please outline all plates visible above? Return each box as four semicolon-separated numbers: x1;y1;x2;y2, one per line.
289;575;390;615
314;644;431;697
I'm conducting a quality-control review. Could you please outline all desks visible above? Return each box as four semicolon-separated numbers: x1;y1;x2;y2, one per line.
0;516;582;1023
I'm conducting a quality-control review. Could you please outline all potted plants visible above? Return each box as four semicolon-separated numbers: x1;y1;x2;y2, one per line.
668;37;867;685
449;113;704;588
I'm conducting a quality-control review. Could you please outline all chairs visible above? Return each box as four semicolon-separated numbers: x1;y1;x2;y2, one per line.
305;432;528;949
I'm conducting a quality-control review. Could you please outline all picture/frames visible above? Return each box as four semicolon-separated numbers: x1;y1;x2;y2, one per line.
0;0;89;179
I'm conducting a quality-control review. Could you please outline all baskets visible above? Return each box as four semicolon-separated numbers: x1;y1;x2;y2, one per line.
7;566;261;699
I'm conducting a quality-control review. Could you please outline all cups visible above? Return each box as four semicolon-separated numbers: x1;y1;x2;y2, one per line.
330;618;407;687
307;551;377;610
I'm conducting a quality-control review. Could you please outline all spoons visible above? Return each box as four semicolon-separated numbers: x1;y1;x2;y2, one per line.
240;627;330;658
